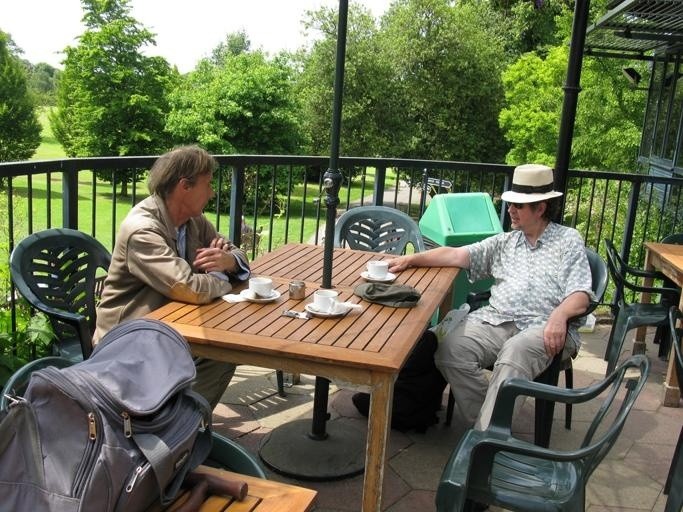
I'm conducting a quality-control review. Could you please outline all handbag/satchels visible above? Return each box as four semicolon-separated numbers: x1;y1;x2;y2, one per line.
353;330;447;433
0;317;215;511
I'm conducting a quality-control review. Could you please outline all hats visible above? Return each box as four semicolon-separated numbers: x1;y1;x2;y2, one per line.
501;164;564;204
356;282;420;308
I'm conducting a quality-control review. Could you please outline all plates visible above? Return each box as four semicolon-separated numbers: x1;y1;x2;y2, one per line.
304;302;347;319
360;271;396;283
240;288;280;304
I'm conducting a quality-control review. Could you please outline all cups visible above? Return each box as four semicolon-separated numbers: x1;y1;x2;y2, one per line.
312;287;340;311
367;261;389;279
250;277;273;295
287;280;307;300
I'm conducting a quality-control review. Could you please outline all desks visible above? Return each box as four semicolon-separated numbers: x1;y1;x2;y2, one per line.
142;241;461;510
154;465;318;510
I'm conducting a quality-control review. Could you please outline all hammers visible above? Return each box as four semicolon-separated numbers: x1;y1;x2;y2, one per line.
166;473;248;512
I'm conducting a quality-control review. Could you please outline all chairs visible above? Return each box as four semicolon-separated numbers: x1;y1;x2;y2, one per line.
3;356;266;481
435;353;651;512
9;229;113;365
333;206;425;255
444;203;683;451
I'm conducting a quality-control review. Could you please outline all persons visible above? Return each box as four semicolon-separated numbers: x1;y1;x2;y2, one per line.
378;163;600;436
89;142;250;417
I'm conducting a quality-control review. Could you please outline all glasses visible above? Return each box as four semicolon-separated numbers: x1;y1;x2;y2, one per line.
507;202;527;209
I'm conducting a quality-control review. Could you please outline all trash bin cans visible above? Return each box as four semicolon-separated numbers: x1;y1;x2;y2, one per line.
418;193;505;329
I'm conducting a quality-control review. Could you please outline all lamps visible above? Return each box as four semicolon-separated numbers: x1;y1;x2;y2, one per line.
622;67;652;91
